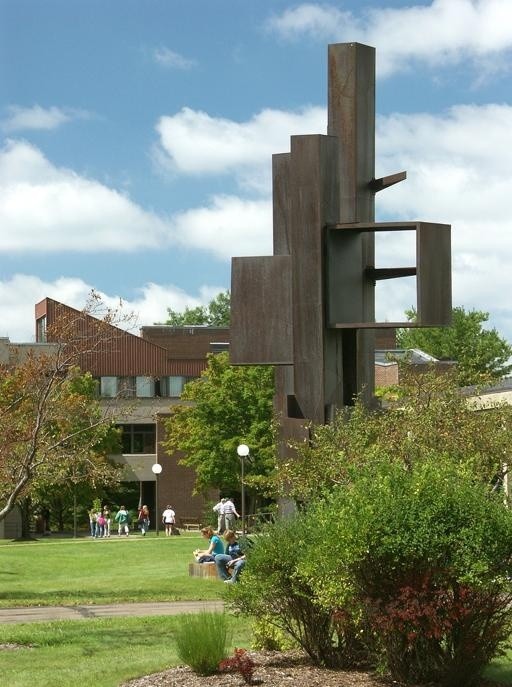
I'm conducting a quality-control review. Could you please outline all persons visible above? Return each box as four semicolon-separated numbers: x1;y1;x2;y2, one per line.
213;530;244;580
223;554;248;584
137;505;150;537
223;498;241;531
212;498;227;535
160;504;176;538
192;526;224;563
86;504;130;539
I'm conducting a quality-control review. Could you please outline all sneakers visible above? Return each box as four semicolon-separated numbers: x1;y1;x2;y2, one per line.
91;534;111;539
224;579;236;585
118;532;147;538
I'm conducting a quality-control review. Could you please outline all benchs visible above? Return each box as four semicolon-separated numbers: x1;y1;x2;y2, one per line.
186;559;237;579
178;515;204;532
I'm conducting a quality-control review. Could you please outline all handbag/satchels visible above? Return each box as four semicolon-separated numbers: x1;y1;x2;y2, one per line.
136;518;147;525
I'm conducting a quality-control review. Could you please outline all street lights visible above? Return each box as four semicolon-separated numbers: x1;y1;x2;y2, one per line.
236;444;250;536
152;463;163;536
